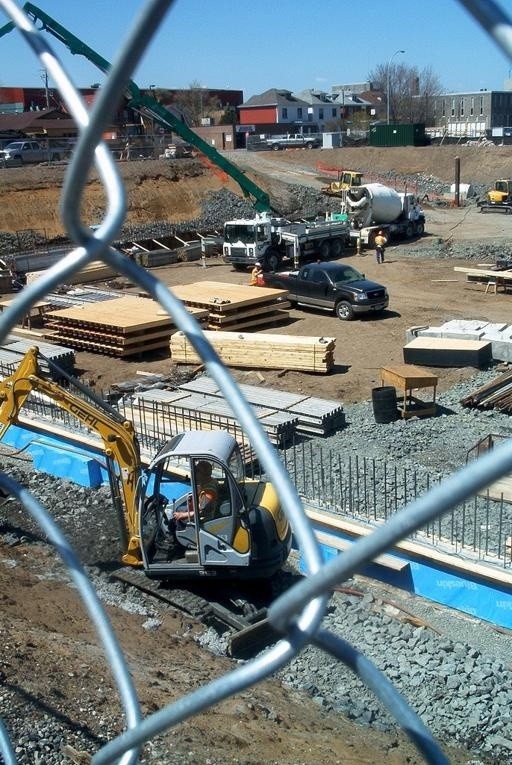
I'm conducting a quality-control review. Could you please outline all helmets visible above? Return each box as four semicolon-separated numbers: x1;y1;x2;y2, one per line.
197;461;212;473
378;231;384;236
255;262;261;267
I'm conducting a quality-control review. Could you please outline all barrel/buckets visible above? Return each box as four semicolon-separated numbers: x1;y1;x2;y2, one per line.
451;183;473;197
371;386;398;424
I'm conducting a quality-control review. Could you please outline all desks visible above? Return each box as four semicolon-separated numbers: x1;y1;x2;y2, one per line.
379;364;439;419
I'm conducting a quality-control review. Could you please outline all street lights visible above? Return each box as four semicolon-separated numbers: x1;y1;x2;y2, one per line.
149;84;157;136
385;48;406;126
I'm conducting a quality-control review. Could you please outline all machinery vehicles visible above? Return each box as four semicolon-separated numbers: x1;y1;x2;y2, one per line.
0;350;296;589
477;179;512;216
321;168;366;197
340;182;429;248
0;1;352;273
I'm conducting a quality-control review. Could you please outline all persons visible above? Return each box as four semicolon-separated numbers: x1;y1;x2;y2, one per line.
249;261;263;286
166;460;222;561
373;230;387;264
257;267;267;287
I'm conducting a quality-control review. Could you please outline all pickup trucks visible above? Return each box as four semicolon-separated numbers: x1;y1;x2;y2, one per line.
264;130;319;151
265;261;391;319
0;140;67;166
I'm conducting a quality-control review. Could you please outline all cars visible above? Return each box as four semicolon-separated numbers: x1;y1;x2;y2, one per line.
110;144;198;162
